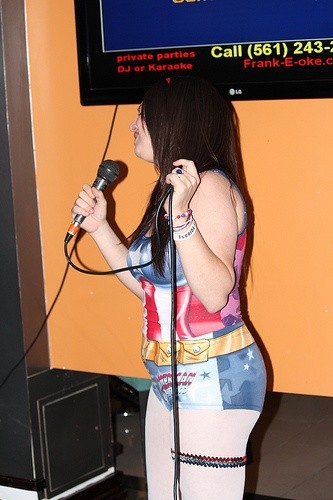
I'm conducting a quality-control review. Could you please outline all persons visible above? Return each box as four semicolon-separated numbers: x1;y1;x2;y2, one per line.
71;73;267;500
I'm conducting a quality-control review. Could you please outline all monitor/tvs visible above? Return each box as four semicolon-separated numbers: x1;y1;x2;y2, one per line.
74;0;333;107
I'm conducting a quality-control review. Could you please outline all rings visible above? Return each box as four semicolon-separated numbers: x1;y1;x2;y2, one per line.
175;169;184;177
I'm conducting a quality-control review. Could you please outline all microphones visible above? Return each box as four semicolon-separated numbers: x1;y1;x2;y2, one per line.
64;160;119;243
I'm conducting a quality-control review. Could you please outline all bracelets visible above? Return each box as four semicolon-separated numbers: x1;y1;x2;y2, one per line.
172;215;193;231
173;219;197;240
164;209;192;220
